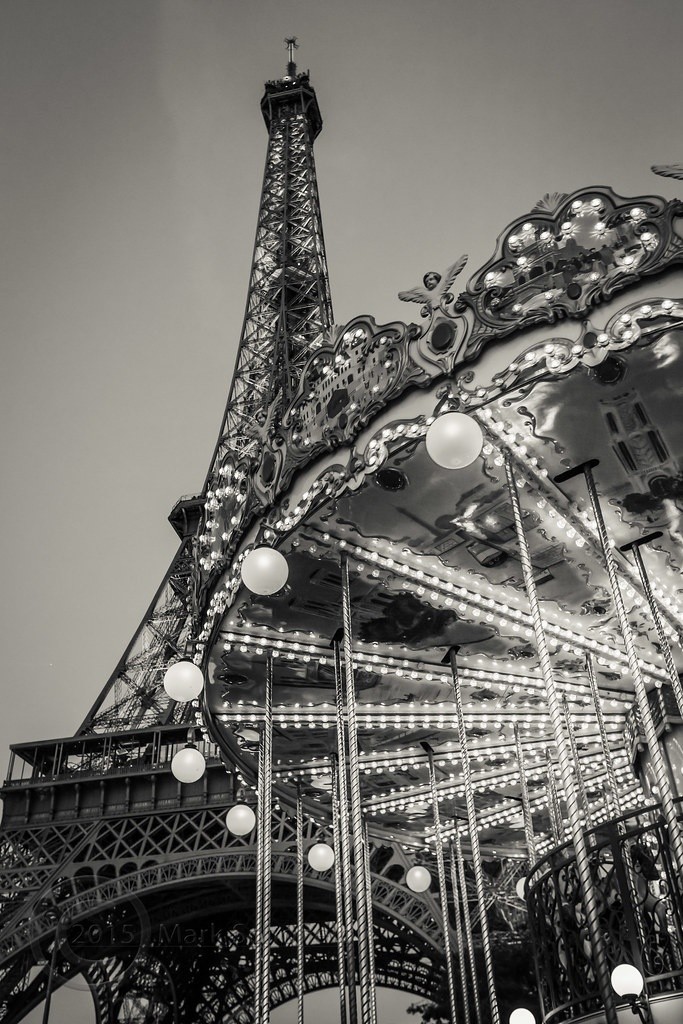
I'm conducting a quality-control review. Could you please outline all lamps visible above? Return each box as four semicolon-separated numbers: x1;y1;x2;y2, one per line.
611;964;654;1024
241;541;289;595
406;865;432;893
516;877;537;902
308;841;335;871
171;743;206;783
426;412;482;470
226;802;256;836
163;658;204;702
509;1008;536;1024
